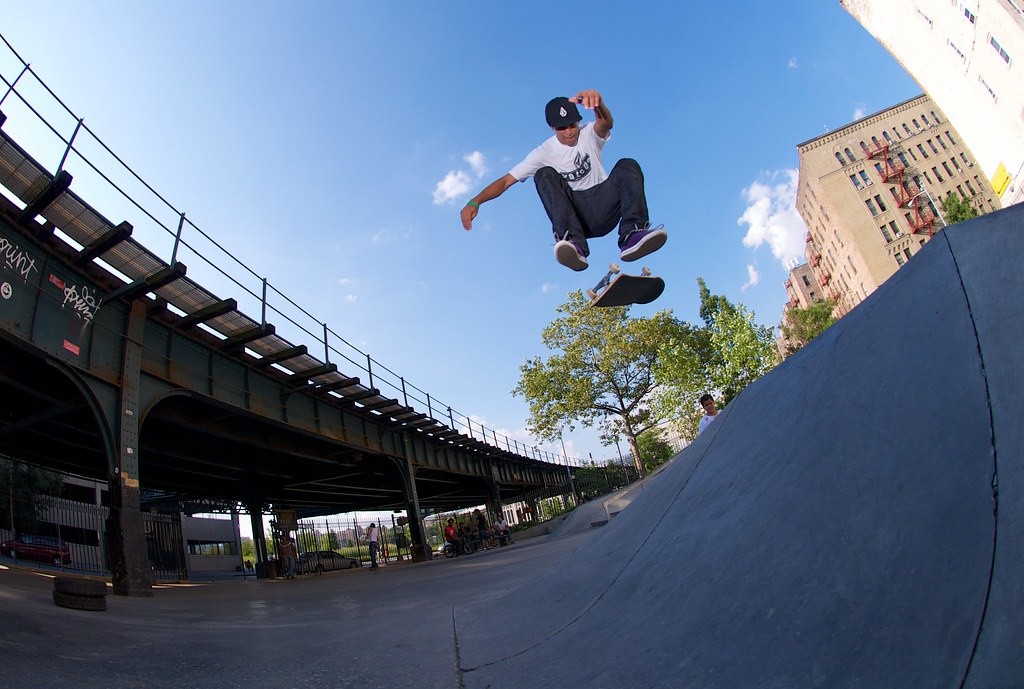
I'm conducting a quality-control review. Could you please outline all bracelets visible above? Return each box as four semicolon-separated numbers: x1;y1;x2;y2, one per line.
467;201;480;209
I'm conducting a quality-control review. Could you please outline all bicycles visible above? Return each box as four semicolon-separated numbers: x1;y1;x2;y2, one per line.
444;526;500;558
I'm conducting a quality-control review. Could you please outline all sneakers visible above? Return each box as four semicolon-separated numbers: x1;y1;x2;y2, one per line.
553;230;589;272
620;220;667;263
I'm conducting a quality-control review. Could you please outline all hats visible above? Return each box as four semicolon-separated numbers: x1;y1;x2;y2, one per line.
545;96;583;129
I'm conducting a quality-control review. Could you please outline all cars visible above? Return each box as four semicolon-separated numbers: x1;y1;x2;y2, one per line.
0;535;72;567
300;551;360;573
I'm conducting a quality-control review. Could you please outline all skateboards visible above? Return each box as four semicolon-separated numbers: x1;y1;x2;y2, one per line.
586;264;666;309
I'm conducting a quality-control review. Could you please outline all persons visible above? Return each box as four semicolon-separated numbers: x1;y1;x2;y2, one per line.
445;518;464;558
458;522;477;552
367;523;380;572
699;394;722;435
460;90;668;272
279;535;296;580
485;516;514;548
473;509;490;551
